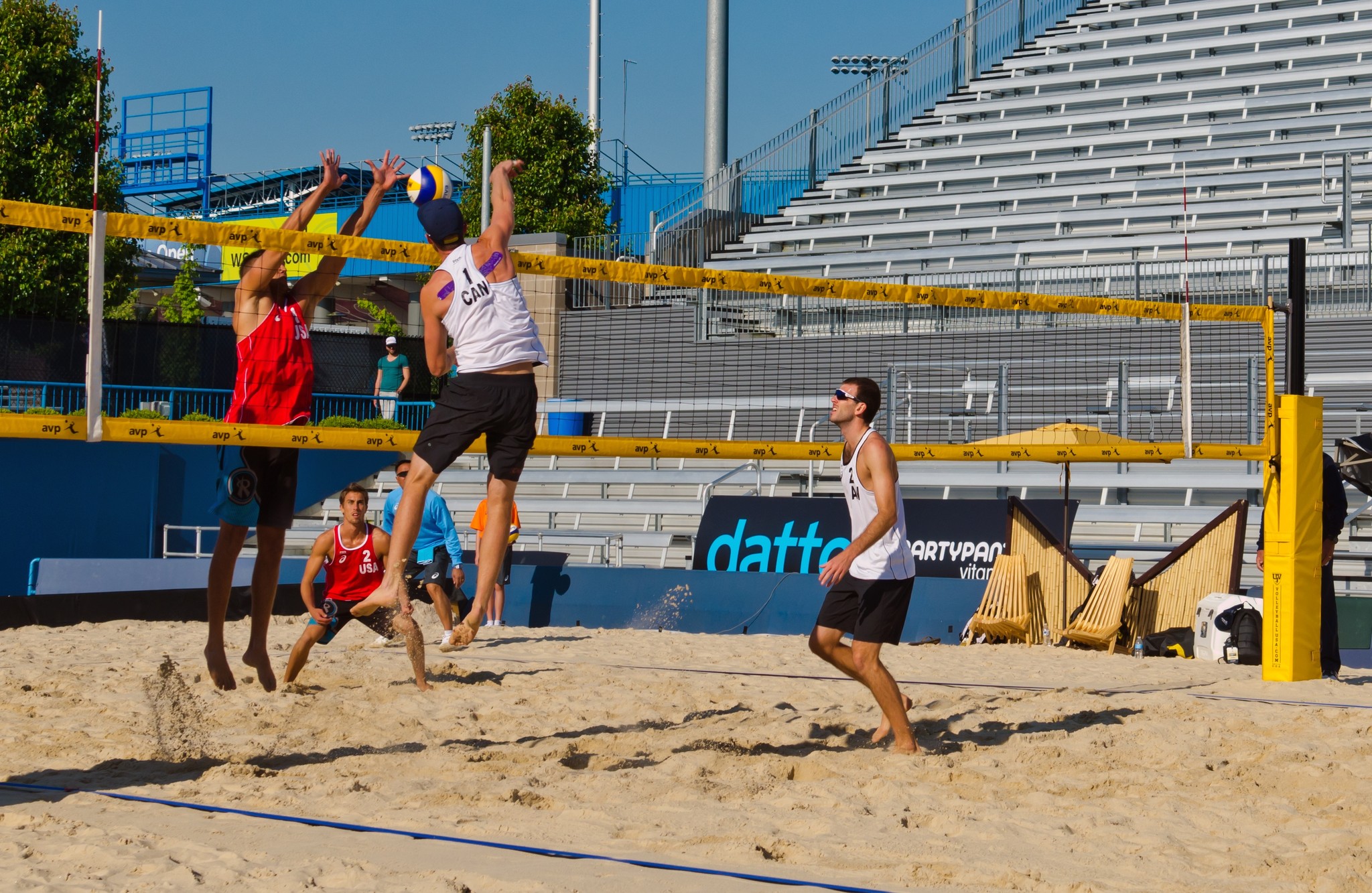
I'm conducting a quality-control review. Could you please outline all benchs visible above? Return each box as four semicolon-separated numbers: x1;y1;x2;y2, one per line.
704;0;1372;271
280;453;1372;595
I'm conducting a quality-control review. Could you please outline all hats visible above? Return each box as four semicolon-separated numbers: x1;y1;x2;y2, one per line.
386;336;397;345
417;198;464;244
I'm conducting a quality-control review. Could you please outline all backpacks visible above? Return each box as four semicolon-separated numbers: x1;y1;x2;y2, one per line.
1223;601;1262;666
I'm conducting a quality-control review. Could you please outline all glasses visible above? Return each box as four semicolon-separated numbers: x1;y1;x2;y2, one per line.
834;388;864;403
397;470;409;477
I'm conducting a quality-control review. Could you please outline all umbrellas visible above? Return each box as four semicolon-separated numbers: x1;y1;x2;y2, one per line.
964;418;1171;631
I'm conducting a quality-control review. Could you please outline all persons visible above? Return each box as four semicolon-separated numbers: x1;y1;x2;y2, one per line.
373;459;466;647
283;483;434;691
469;498;522;626
1255;452;1349;679
807;377;922;755
373;336;410;420
203;148;411;693
350;160;550;649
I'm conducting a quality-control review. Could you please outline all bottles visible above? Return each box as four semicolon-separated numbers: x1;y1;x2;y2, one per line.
1042;623;1051;647
1135;636;1143;658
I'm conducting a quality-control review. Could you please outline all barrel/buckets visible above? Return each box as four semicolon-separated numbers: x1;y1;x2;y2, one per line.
547;399;593;437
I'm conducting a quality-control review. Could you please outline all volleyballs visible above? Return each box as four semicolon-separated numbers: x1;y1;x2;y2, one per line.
406;165;454;208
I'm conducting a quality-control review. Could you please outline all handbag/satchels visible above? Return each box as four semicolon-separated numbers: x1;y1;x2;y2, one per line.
1068;604;1129;647
1131;626;1195;659
1214;604;1244;631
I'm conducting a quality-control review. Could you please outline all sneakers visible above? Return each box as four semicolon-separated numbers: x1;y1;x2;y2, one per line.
375;633;404;646
1322;668;1338;680
438;633;463;652
